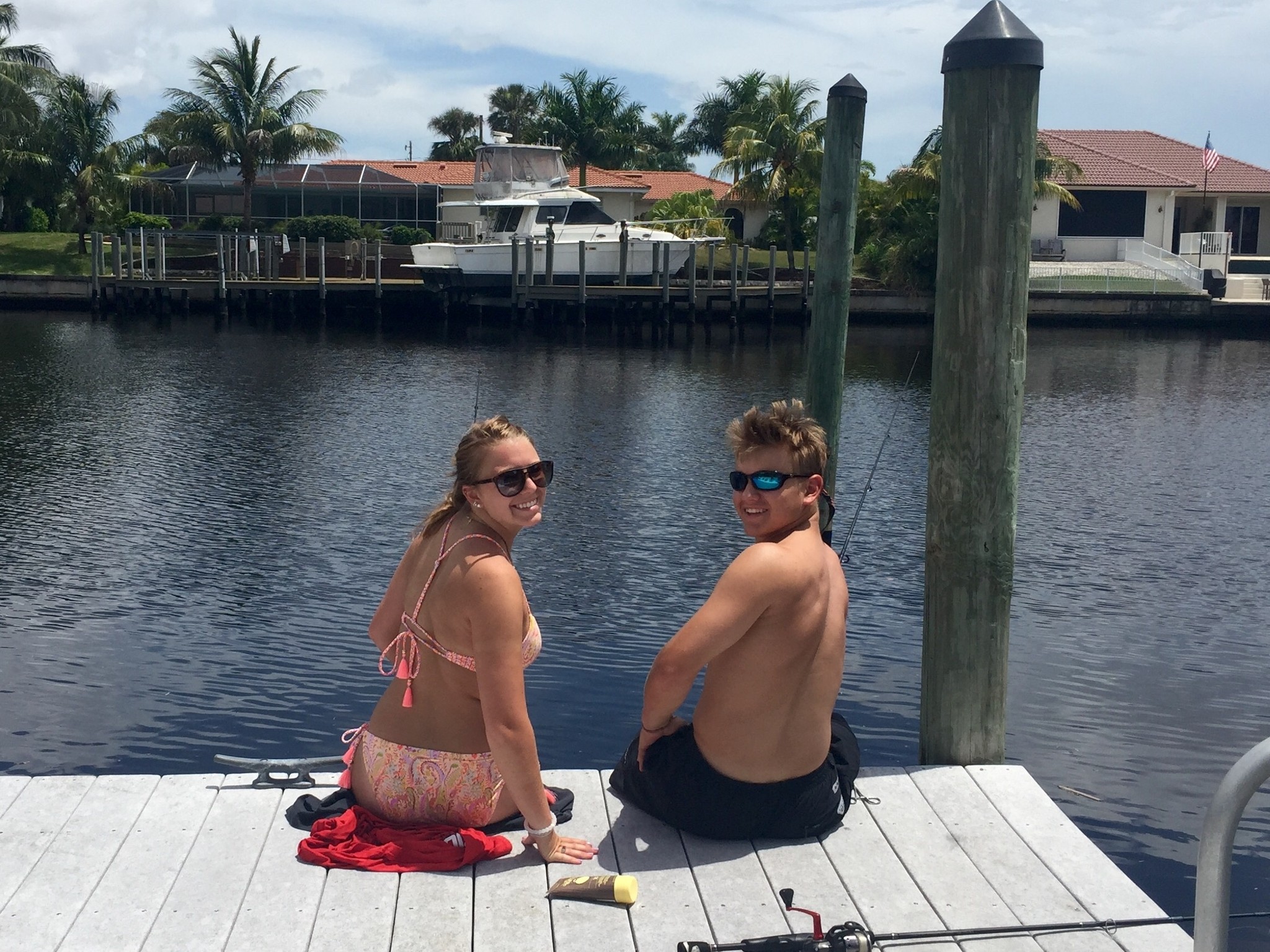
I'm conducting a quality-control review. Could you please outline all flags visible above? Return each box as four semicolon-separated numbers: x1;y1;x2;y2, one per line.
1203;135;1220;174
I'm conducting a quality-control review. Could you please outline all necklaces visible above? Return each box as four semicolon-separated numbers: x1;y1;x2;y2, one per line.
466;515;513;563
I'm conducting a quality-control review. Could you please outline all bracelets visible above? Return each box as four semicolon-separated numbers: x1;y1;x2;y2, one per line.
642;715;675;732
523;811;557;837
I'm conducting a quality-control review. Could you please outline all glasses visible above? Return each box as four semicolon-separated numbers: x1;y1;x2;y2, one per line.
730;470;813;491
470;461;554;498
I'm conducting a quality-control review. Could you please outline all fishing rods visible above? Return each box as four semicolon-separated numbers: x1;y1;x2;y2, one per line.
473;368;484;423
836;348;922;564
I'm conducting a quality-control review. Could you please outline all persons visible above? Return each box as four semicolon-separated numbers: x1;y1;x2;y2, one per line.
339;415;599;864
613;400;860;840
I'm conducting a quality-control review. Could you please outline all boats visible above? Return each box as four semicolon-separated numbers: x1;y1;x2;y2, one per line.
397;114;735;293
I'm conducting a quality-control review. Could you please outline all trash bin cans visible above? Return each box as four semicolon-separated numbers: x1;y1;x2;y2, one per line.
1203;269;1227;300
